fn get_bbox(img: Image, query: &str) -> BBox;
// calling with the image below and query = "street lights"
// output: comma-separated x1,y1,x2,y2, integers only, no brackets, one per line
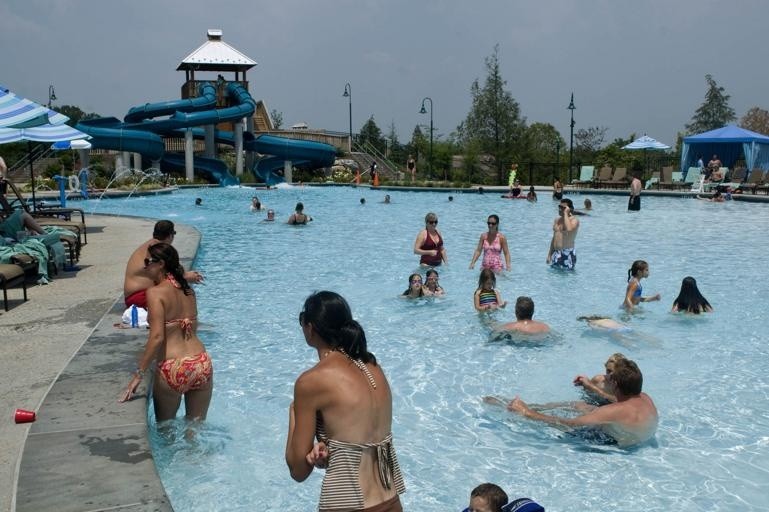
48,84,58,106
566,92,576,185
343,83,352,154
418,97,433,181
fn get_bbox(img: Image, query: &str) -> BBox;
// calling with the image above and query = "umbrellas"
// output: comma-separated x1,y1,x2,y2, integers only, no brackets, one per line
620,133,673,188
0,85,95,217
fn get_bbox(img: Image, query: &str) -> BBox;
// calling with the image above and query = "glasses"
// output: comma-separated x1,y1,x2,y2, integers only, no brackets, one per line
144,258,157,267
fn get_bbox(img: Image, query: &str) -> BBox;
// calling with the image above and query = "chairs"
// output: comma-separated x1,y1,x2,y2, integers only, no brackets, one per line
0,176,89,314
569,164,769,196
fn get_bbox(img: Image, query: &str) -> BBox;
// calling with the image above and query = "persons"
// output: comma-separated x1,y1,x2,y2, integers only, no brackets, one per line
550,175,564,201
501,161,539,203
576,311,656,354
1,156,14,213
384,194,391,203
400,195,599,341
119,214,215,444
463,481,511,512
627,172,643,212
194,198,202,205
406,153,417,182
619,259,662,316
2,204,49,242
478,353,660,454
670,273,716,317
477,186,484,195
247,197,313,226
283,288,409,512
695,153,735,203
369,161,379,186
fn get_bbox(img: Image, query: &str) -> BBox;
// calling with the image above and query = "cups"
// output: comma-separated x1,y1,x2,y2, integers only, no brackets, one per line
14,409,36,422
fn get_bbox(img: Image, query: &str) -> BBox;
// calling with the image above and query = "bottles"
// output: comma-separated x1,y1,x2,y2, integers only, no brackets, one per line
131,305,139,327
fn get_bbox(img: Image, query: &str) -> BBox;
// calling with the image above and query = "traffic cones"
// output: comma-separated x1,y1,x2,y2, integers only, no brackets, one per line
374,173,379,186
